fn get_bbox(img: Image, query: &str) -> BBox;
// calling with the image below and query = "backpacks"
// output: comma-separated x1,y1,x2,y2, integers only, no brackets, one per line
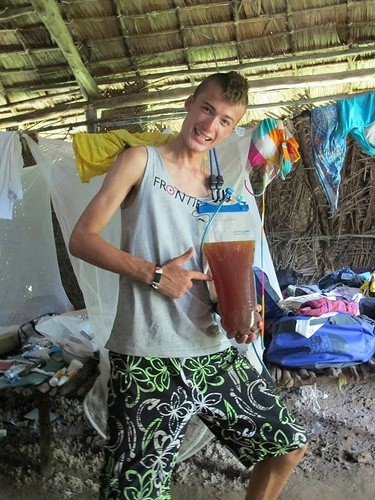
254,267,375,368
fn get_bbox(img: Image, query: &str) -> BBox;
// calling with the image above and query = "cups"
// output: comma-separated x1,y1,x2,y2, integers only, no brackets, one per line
67,359,84,373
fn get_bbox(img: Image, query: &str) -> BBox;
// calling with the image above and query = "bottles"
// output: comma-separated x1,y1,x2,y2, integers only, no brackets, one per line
57,369,78,387
49,367,66,386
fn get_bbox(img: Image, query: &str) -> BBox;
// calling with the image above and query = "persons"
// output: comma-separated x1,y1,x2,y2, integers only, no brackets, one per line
67,70,313,500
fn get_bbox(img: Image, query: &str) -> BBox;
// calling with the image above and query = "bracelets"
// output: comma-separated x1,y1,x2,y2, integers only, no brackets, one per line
149,264,162,290
